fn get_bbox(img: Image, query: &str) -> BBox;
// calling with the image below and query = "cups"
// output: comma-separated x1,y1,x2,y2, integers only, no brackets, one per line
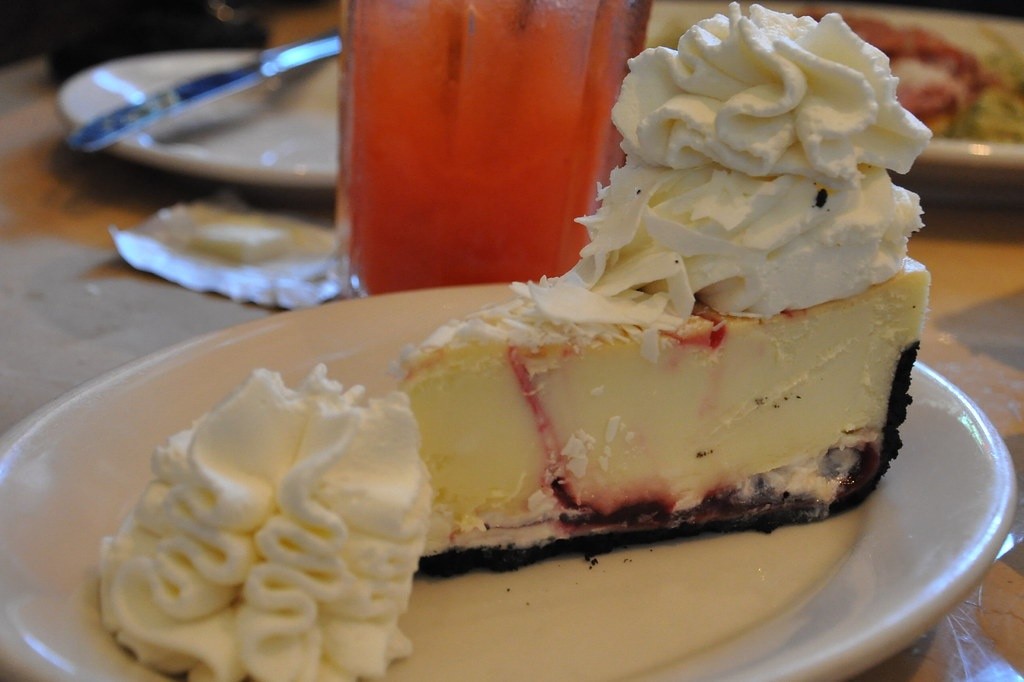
340,0,642,296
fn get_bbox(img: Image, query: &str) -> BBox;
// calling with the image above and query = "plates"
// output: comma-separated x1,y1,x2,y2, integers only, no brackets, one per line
647,0,1024,168
58,52,341,187
0,284,1015,682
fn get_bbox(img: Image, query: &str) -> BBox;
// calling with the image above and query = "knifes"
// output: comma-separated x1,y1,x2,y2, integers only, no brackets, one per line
68,34,341,153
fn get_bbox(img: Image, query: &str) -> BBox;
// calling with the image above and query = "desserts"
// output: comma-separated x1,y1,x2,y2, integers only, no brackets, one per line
97,1,933,682
